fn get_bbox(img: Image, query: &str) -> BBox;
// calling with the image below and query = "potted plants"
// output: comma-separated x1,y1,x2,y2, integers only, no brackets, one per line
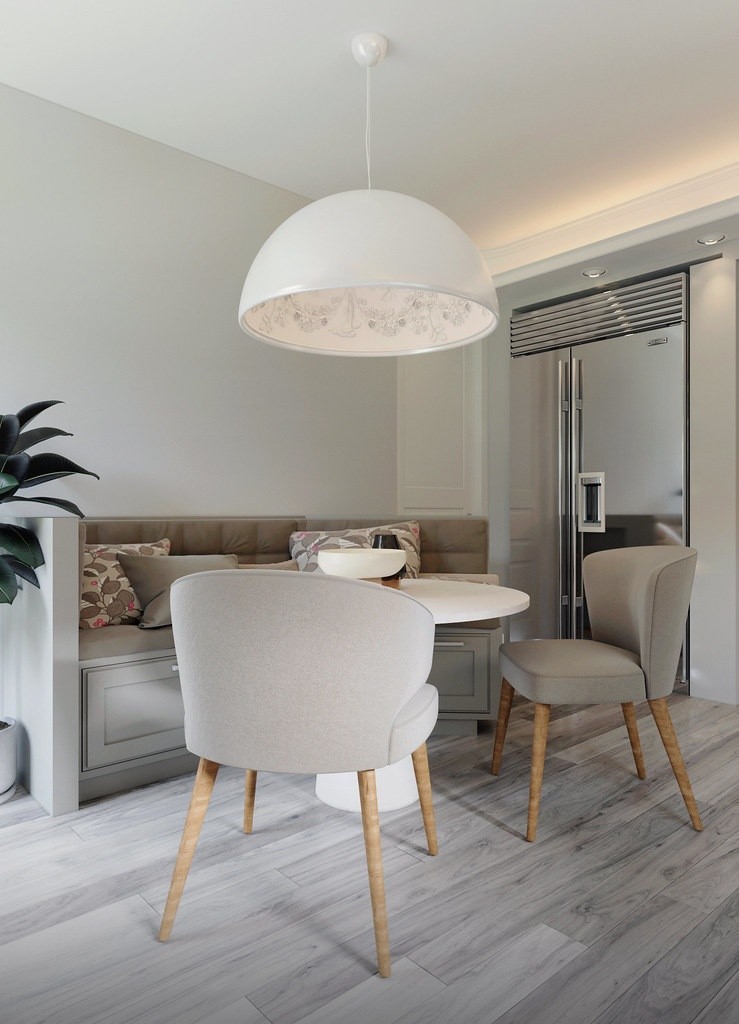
0,400,100,807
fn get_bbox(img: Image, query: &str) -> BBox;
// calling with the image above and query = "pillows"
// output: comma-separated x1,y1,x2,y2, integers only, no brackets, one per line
238,558,299,570
289,519,421,580
79,537,171,630
116,553,239,630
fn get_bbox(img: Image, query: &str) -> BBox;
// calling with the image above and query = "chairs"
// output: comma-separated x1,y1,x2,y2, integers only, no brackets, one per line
154,568,437,977
490,546,704,843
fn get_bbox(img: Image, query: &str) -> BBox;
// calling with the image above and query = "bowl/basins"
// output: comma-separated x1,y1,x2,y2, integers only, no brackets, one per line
317,547,408,579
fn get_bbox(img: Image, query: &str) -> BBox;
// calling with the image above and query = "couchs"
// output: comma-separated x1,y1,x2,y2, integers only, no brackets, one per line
15,514,503,817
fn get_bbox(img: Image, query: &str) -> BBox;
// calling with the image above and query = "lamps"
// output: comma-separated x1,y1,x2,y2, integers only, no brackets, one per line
237,34,500,357
